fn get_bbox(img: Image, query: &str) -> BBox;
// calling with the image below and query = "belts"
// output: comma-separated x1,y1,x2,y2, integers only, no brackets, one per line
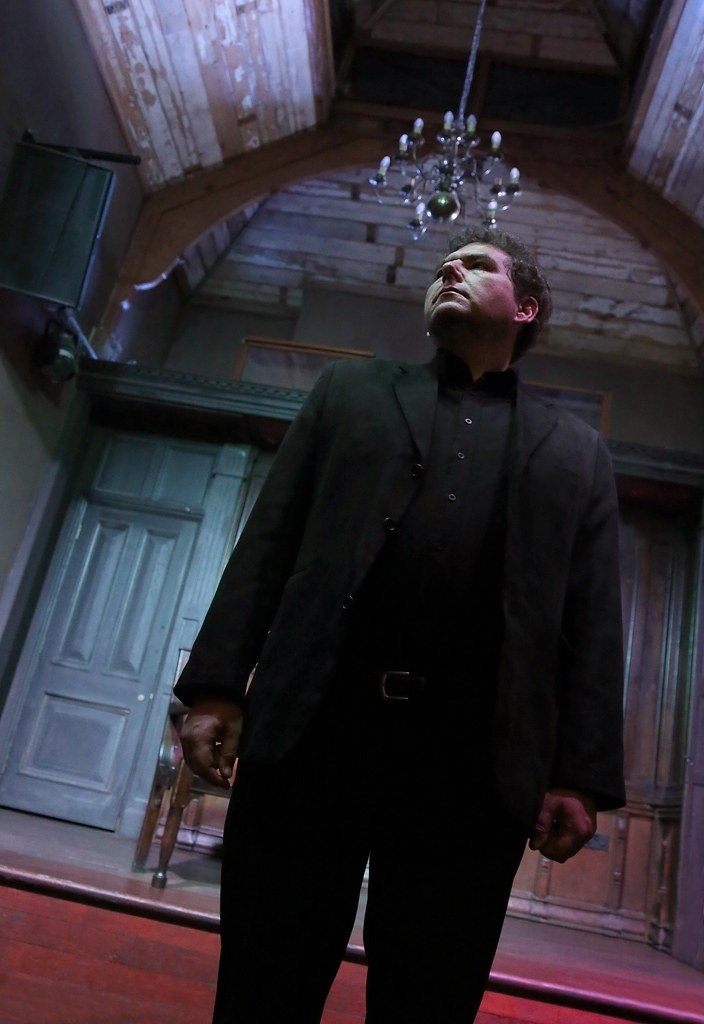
326,667,495,706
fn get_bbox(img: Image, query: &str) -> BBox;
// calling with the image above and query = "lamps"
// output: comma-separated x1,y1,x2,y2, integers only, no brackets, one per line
366,0,525,239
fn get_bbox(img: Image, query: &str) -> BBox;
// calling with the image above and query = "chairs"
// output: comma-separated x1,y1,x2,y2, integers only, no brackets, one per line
129,647,234,890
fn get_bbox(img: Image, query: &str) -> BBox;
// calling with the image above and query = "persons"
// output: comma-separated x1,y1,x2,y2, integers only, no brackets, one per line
174,229,626,1023
